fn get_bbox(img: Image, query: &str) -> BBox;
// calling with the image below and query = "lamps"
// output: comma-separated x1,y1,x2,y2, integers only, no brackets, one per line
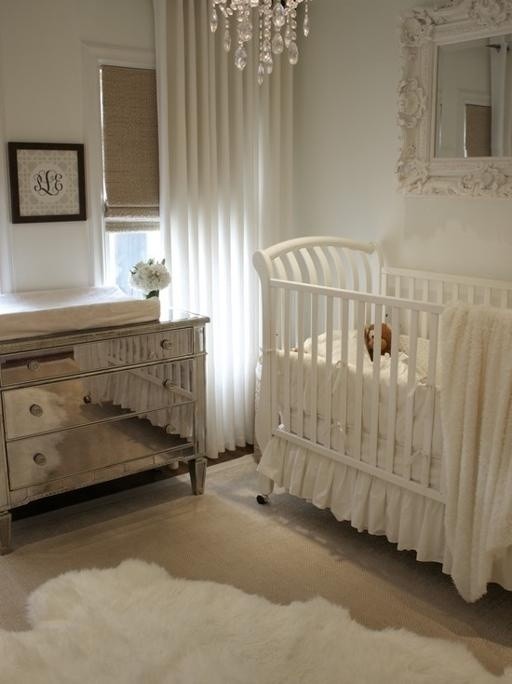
204,0,312,89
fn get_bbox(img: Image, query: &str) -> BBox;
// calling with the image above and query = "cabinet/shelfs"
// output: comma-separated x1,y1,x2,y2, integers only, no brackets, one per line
1,308,207,554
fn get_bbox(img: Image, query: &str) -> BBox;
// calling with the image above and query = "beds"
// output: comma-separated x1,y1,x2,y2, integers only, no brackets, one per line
251,238,511,601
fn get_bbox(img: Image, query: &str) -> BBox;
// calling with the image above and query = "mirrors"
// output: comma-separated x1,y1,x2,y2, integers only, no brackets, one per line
396,0,511,202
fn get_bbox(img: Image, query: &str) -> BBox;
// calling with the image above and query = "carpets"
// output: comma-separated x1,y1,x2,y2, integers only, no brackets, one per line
0,494,512,684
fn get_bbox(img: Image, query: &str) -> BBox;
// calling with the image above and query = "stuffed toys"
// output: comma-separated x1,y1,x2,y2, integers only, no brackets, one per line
366,323,422,384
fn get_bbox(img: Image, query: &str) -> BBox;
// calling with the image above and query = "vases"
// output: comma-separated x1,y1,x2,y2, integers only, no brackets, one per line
146,291,159,301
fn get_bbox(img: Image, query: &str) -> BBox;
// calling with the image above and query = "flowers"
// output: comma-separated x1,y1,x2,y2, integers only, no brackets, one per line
130,256,171,296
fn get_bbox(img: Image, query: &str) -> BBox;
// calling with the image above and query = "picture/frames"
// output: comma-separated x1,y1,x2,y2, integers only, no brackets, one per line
8,143,86,225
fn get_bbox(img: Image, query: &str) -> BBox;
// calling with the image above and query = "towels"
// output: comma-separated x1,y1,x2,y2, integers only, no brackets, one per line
433,301,511,601
